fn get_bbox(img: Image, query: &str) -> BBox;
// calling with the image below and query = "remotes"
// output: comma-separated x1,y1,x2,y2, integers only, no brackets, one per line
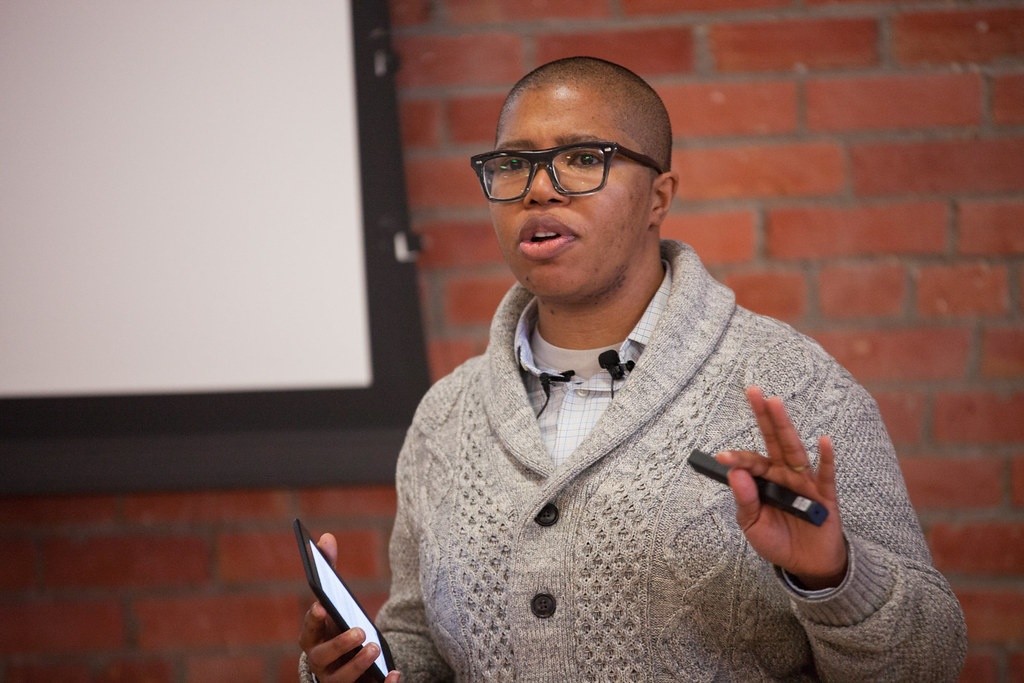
687,448,829,526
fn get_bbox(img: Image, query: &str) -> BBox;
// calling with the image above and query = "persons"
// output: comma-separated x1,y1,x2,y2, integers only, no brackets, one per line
300,55,970,683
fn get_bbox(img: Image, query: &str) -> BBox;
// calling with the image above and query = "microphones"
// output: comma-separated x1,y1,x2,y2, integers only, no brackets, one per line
598,349,625,380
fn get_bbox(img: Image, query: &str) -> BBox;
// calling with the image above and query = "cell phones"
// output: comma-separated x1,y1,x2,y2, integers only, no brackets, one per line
293,518,395,683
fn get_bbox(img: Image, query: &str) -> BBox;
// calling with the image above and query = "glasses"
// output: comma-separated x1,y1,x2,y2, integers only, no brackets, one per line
470,142,666,204
